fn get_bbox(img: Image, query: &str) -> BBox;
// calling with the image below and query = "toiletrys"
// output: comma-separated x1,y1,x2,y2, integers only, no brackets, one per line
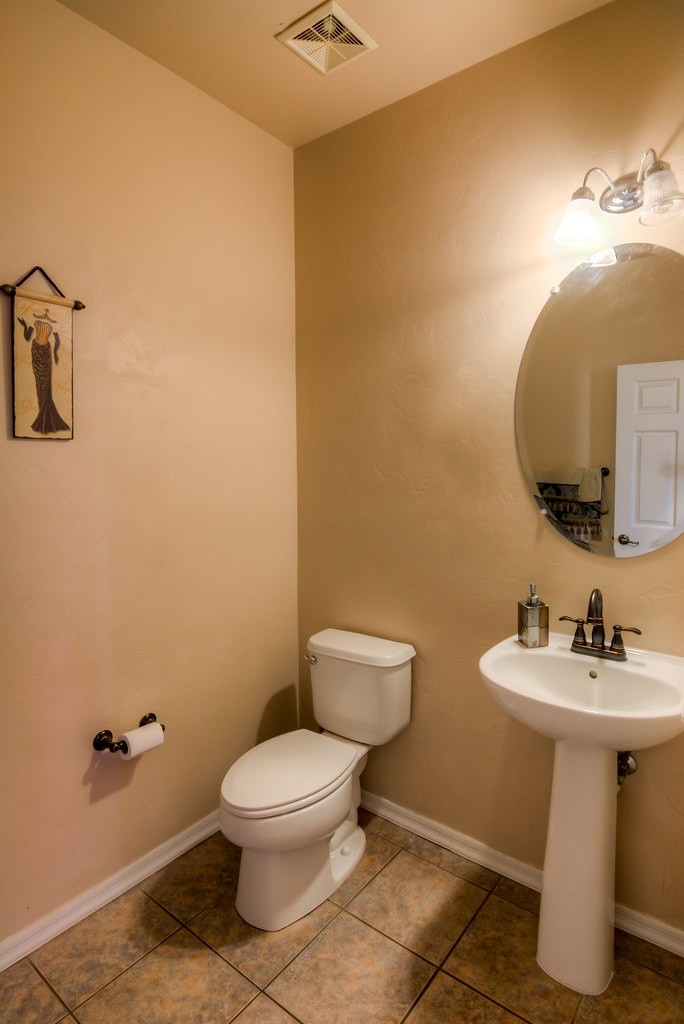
518,584,549,648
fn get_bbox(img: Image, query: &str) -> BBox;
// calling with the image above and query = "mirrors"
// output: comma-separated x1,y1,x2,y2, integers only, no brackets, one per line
513,242,683,560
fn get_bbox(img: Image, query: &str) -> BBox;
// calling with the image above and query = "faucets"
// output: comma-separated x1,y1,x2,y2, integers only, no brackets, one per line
557,588,641,662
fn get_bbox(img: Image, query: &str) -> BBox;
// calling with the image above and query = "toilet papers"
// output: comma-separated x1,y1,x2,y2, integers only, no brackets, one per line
119,721,165,759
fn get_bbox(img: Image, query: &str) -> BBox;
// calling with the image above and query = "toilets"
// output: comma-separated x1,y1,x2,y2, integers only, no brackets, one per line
219,630,415,931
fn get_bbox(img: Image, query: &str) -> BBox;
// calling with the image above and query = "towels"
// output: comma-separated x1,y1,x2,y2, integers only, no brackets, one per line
578,467,610,515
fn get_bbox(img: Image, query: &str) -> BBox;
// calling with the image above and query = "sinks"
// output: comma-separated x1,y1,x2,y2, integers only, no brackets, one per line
478,629,684,750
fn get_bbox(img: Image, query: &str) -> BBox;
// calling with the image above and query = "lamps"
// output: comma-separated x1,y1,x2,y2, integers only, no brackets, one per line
552,148,684,246
